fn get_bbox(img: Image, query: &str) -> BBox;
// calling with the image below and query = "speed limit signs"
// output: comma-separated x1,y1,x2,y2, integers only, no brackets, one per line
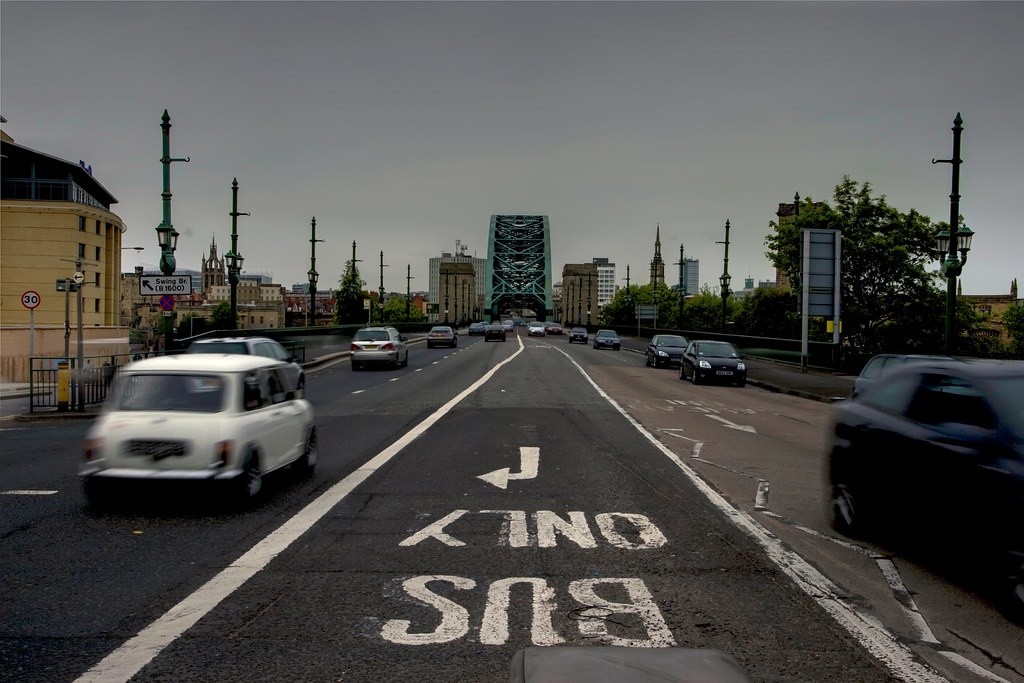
22,291,41,309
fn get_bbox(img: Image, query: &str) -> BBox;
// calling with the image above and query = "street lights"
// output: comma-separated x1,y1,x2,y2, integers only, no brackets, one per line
676,241,685,329
577,278,583,326
225,178,248,332
570,282,575,325
653,258,662,330
442,267,450,324
623,263,633,327
718,219,731,333
564,286,571,326
454,274,459,326
378,249,389,322
586,271,592,327
348,240,362,319
460,280,467,324
305,214,325,328
466,284,473,320
404,264,414,322
785,192,803,343
154,107,181,354
931,112,976,355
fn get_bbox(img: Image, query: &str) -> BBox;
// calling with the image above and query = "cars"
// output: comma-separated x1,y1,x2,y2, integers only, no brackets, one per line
427,325,457,349
545,322,563,336
845,353,967,400
483,324,507,342
819,360,1024,630
678,340,748,388
187,334,309,401
469,319,528,336
644,335,690,367
527,321,545,336
78,351,320,508
593,329,621,351
349,325,410,370
567,327,589,345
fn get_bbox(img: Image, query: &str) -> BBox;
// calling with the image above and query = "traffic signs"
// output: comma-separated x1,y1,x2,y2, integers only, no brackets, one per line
137,274,193,295
